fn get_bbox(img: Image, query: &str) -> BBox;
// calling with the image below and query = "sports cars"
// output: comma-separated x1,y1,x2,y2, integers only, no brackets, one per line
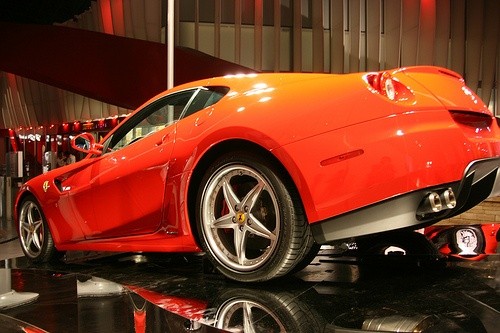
424,224,500,261
68,269,323,333
11,64,500,286
